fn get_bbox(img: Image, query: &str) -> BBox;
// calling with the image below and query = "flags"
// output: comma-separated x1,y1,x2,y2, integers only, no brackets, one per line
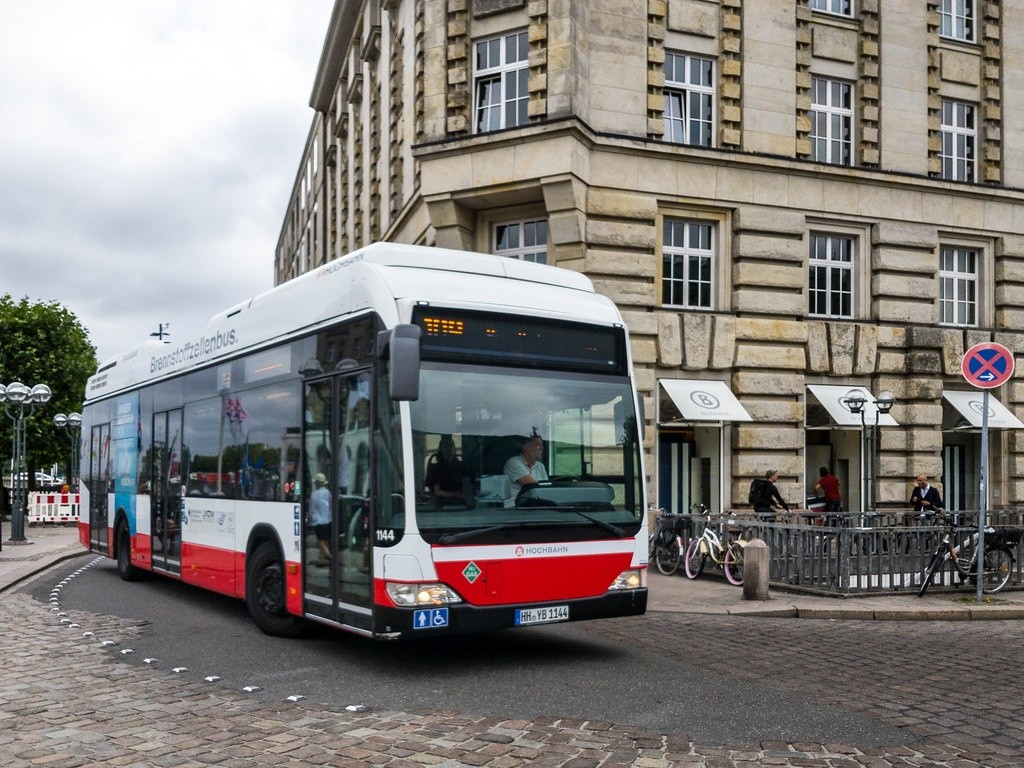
236,397,263,438
226,398,239,439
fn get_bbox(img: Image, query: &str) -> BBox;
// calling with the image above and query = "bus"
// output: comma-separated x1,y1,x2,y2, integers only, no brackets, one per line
80,241,649,641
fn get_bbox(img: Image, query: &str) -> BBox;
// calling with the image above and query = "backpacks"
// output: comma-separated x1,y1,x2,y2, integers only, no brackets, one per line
748,478,765,504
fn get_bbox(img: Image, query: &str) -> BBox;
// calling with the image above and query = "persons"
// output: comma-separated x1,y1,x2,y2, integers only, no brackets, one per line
307,472,333,561
432,436,473,497
814,466,842,527
502,433,549,508
909,474,943,521
753,469,793,522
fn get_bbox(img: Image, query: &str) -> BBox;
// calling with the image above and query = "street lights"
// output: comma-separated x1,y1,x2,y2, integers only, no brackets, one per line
0,382,52,546
843,390,895,555
53,412,82,493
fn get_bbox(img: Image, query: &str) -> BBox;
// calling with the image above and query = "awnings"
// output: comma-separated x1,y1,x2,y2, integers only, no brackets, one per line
657,378,754,422
805,385,901,426
942,390,1024,428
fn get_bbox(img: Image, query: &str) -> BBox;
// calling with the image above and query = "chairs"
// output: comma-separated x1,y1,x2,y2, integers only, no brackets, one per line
425,423,524,506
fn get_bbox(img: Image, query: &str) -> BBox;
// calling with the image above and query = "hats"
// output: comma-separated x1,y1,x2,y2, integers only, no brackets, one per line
765,468,777,480
312,473,328,485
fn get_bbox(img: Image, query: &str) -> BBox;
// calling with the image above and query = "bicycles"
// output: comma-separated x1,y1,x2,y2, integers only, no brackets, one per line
649,508,691,576
685,502,748,586
917,505,1016,598
